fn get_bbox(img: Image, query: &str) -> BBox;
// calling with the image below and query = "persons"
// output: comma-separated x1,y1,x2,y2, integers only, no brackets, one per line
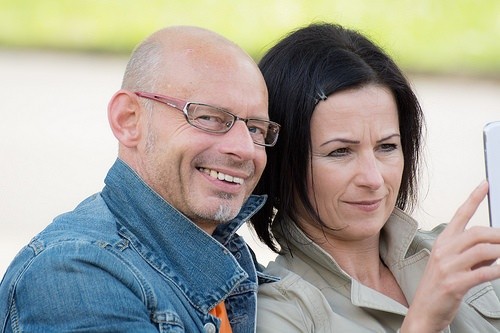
254,19,500,333
0,25,280,333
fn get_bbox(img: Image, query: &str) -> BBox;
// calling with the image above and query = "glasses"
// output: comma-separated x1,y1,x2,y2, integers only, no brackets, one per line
131,88,282,149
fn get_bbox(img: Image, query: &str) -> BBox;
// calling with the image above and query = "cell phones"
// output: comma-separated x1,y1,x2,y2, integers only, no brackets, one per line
483,121,500,231
